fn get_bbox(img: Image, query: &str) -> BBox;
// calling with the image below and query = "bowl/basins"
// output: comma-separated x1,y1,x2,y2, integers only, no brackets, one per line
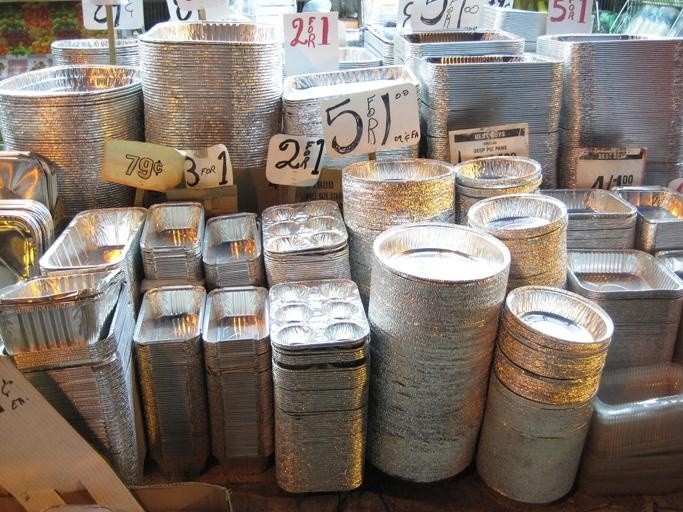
475,285,613,504
39,206,146,315
454,155,542,226
204,287,273,466
140,202,204,281
133,285,211,473
202,212,263,288
343,158,457,316
366,221,511,483
468,193,567,289
0,269,145,484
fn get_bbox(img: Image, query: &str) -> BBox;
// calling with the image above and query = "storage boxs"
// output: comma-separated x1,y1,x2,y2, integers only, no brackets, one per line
0,482,232,512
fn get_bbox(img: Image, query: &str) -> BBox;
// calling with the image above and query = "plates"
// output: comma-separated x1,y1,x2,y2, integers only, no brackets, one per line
569,247,683,367
0,151,63,237
586,365,683,496
140,21,284,169
284,64,422,168
365,27,394,66
542,188,637,249
261,200,350,288
52,37,140,67
0,62,145,230
394,27,526,157
340,47,382,71
267,280,370,493
479,4,548,52
613,185,682,253
657,250,682,280
421,53,565,188
0,199,53,286
537,33,683,190
